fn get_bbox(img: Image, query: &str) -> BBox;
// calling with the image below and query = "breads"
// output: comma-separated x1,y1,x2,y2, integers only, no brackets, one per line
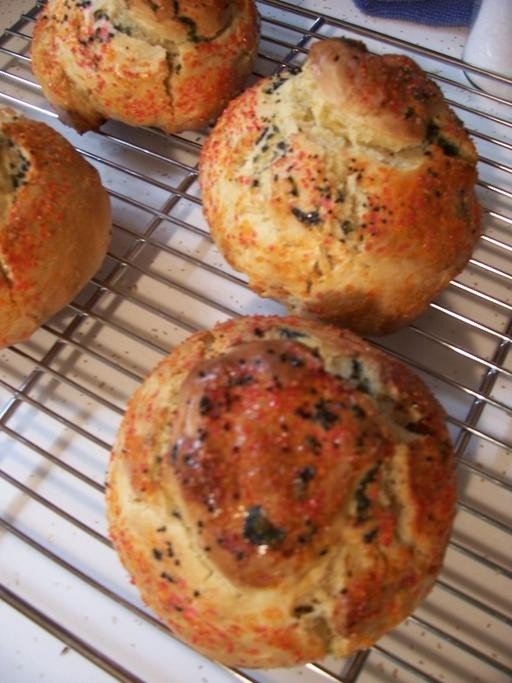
0,105,112,351
31,0,261,136
199,39,485,337
106,314,457,668
